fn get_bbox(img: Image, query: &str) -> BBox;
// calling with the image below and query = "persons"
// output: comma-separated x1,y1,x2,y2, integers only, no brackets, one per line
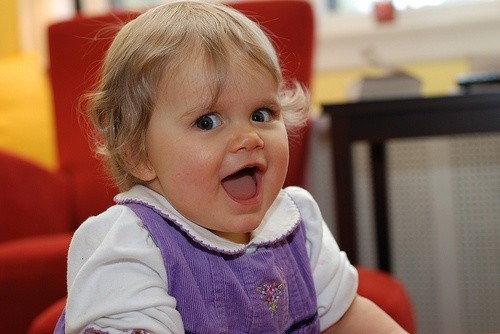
53,0,414,334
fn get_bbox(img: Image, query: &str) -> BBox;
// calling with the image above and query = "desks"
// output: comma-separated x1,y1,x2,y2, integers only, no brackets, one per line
320,92,500,275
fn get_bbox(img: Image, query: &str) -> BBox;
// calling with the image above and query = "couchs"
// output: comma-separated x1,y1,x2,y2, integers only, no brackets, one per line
0,1,416,334
